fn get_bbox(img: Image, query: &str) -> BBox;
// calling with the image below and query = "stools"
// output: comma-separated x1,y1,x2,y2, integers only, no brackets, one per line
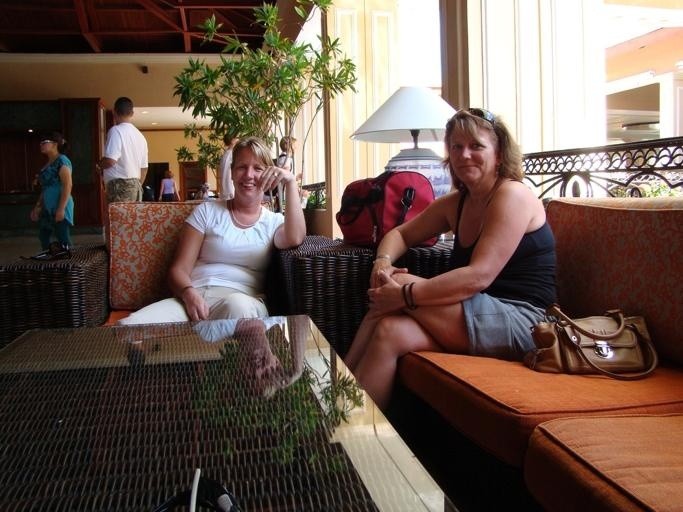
0,243,109,352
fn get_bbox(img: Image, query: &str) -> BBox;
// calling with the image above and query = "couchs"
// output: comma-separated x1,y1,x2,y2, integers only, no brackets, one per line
104,200,339,328
289,194,682,512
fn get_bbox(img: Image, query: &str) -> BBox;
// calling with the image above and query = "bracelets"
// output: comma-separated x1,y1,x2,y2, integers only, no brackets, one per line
402,283,418,311
409,281,416,306
375,255,391,260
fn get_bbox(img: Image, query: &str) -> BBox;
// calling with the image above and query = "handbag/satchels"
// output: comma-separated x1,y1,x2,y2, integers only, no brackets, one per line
334,169,440,249
527,302,659,382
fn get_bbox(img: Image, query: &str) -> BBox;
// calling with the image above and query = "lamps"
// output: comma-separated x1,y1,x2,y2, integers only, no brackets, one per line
348,84,456,243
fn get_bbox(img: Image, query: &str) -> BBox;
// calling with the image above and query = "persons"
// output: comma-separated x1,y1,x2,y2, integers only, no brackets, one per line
94,95,149,204
127,341,147,364
114,136,308,328
340,107,557,407
141,134,296,202
29,131,76,253
193,315,310,398
